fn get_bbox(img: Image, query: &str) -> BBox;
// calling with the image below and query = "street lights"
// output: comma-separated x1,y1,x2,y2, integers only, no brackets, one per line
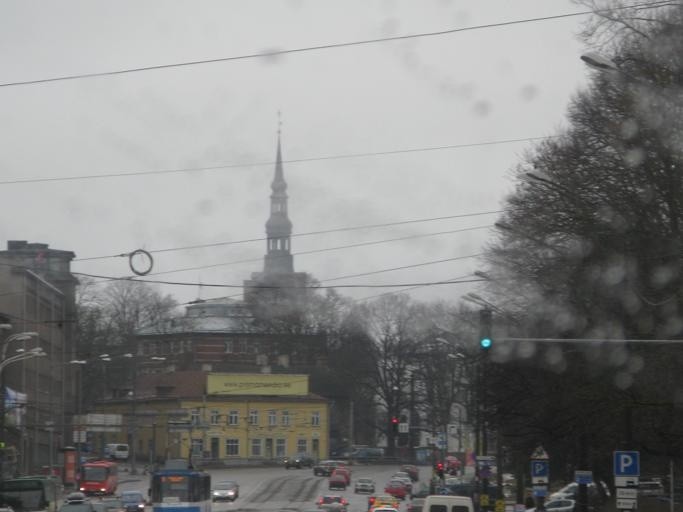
0,323,168,421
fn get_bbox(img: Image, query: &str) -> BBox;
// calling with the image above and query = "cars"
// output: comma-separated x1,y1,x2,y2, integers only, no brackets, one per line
327,468,350,489
523,478,609,511
56,490,146,511
282,452,315,468
444,455,460,469
211,480,239,501
314,494,349,512
354,478,376,493
163,458,194,469
367,464,424,511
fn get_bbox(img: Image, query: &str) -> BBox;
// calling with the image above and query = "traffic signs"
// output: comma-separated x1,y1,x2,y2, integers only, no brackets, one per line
474,454,497,462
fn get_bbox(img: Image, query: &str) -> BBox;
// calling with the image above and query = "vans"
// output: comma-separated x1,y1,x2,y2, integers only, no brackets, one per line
422,495,473,512
637,482,664,496
38,466,60,476
330,442,384,462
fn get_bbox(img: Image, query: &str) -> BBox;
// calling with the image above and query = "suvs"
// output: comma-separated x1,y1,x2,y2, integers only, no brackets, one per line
312,460,344,476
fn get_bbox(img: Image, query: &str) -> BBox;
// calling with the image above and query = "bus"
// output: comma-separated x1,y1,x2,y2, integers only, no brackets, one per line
148,468,211,511
102,442,130,459
78,460,117,497
0,473,60,512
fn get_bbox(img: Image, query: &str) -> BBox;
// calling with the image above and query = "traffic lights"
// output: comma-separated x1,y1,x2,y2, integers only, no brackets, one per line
432,461,445,483
389,413,408,434
